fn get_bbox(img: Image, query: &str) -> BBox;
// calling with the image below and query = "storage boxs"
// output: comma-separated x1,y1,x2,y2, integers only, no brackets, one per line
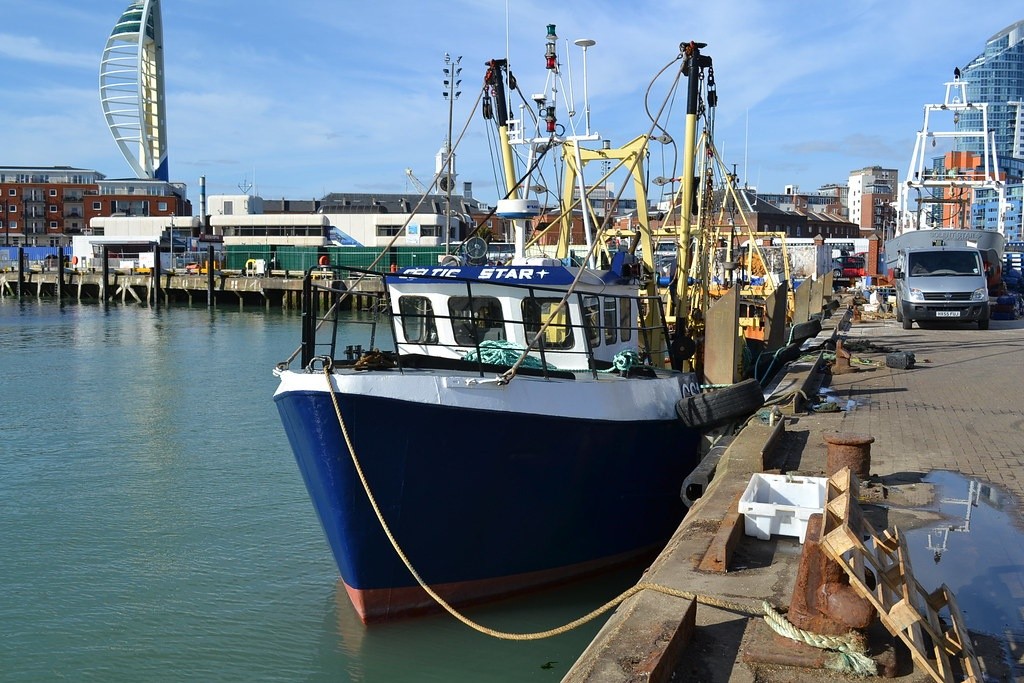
738,473,828,545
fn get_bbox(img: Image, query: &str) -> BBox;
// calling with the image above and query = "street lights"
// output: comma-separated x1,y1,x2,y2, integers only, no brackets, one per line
442,53,462,255
602,161,611,220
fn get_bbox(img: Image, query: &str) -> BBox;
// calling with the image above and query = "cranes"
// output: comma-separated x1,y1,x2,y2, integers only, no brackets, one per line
406,168,433,195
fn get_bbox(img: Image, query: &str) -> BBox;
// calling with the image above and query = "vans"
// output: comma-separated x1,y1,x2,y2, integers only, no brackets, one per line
893,246,990,330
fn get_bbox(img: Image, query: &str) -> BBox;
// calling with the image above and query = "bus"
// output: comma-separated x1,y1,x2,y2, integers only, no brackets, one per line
740,238,870,262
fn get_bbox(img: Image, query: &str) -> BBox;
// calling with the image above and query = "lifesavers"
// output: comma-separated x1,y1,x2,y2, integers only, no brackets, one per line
319,255,330,265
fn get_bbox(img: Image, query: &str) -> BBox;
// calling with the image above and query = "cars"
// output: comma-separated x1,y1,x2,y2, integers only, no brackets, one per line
654,241,677,259
832,256,865,278
840,252,869,276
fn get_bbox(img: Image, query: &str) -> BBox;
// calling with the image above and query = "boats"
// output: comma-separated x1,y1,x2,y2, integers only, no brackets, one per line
886,67,1006,287
272,23,795,624
